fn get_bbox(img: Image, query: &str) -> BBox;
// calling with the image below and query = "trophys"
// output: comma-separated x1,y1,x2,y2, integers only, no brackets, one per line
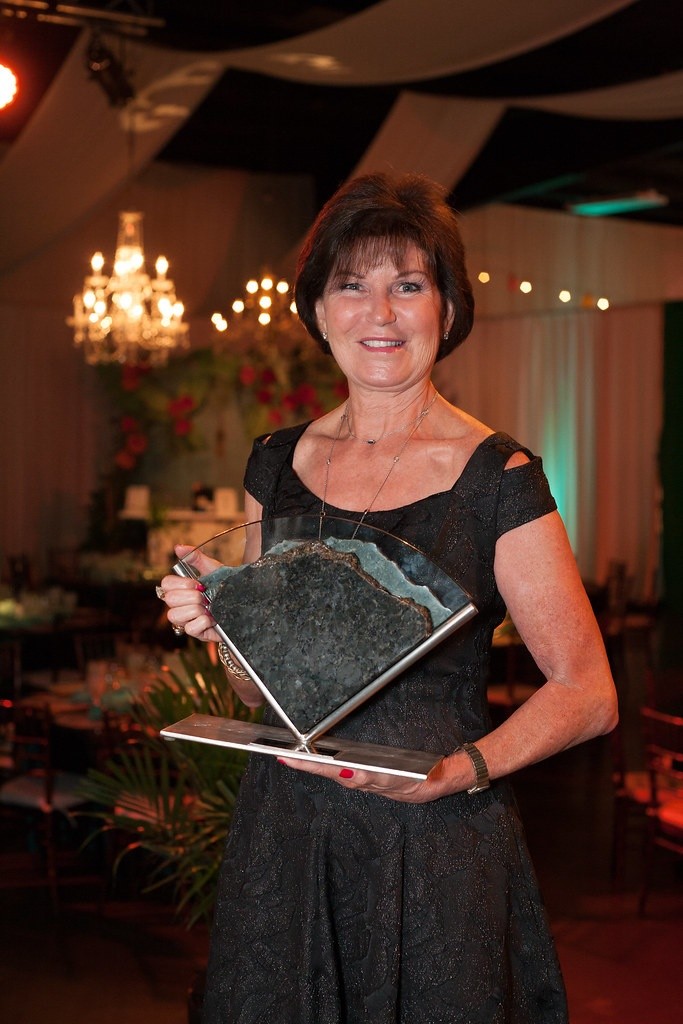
160,514,480,781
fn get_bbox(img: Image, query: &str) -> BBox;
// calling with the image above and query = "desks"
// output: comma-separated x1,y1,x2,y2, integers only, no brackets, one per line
24,681,256,802
0,607,110,688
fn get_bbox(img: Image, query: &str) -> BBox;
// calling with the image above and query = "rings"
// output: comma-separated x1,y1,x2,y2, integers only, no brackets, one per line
155,586,166,604
171,623,186,636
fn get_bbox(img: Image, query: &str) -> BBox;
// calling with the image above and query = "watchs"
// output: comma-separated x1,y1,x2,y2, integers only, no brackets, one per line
452,742,491,795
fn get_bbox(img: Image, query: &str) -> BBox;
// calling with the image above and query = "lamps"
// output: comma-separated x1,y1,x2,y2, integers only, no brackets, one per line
69,111,191,368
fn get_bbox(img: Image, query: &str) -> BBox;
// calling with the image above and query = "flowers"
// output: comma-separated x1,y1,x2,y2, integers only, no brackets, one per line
112,366,195,474
238,368,348,425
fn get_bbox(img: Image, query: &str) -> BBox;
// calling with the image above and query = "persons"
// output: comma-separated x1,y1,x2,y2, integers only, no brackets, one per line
159,173,621,1023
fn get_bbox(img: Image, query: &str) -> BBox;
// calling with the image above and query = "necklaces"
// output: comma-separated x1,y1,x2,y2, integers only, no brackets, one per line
317,390,439,543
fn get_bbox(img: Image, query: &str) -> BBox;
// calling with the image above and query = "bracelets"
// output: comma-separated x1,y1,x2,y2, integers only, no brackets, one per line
216,641,253,682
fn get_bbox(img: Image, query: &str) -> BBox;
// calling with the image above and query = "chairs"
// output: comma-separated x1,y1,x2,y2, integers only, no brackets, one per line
636,706,683,922
0,700,101,878
615,600,657,667
9,555,32,598
